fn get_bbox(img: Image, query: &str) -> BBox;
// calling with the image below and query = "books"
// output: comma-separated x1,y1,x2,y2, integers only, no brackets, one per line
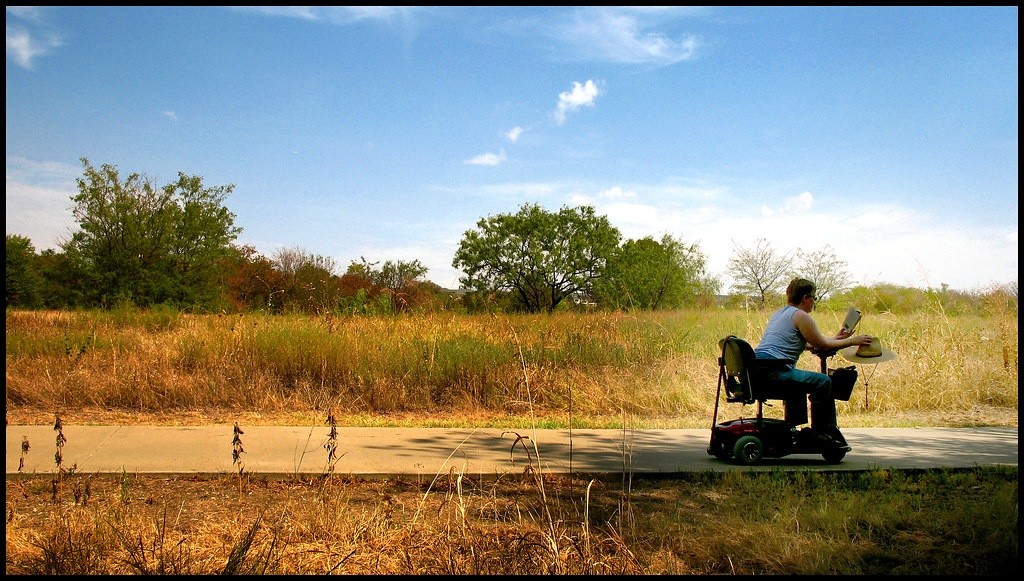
842,309,862,333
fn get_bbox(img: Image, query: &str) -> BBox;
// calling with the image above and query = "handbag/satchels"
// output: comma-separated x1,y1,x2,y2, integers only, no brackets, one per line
830,366,859,401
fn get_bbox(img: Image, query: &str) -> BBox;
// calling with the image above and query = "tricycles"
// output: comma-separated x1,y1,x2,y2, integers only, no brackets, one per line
708,336,849,464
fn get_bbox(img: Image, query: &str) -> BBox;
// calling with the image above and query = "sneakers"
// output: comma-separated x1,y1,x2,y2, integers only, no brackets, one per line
816,432,842,448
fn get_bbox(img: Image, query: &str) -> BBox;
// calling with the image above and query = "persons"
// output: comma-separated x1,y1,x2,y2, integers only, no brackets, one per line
753,279,873,445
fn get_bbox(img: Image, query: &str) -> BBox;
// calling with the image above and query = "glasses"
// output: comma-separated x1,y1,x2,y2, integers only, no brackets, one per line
804,293,819,304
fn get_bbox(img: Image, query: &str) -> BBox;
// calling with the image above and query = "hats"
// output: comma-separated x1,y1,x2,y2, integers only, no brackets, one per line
841,337,898,364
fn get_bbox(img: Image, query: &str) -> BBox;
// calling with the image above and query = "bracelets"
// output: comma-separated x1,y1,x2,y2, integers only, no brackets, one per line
850,338,853,344
